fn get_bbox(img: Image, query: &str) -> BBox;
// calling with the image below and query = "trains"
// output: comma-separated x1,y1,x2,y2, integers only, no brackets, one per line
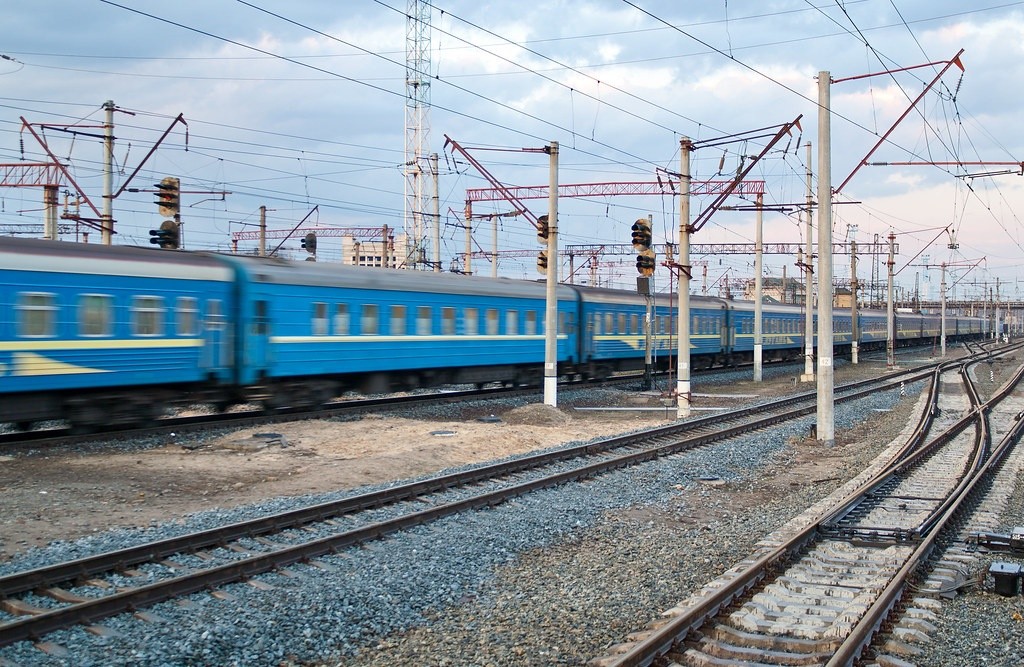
1,232,1007,437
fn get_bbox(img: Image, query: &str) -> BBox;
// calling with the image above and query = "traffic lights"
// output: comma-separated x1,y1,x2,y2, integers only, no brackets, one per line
300,234,317,253
537,249,548,275
154,176,179,218
537,215,549,245
636,247,656,276
631,218,651,252
149,220,179,249
